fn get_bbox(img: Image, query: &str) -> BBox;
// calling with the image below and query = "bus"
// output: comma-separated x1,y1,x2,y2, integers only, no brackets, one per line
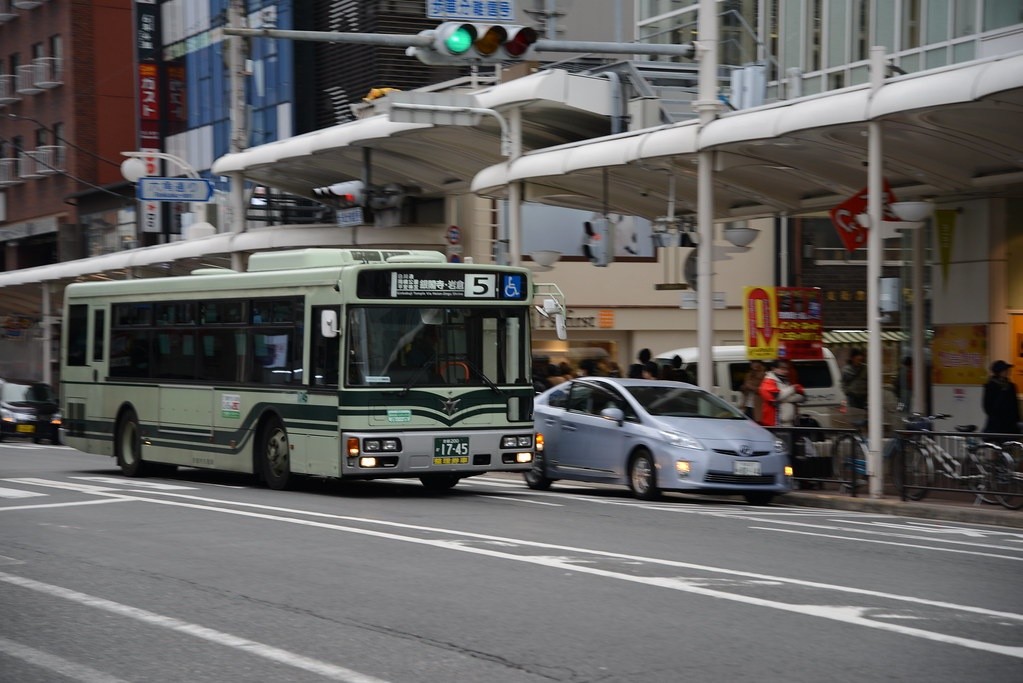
54,248,568,491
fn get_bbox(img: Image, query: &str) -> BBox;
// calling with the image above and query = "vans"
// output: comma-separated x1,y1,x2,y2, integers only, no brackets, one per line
643,343,851,479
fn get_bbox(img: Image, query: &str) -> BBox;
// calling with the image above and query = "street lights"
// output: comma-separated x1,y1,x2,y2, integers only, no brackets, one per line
116,147,218,238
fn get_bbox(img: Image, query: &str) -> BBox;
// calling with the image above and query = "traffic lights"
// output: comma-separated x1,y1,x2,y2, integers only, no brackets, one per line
581,217,617,268
432,20,540,63
312,179,364,205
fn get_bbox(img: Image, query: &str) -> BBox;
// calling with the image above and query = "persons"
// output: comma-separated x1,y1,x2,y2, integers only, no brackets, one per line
980,360,1023,447
740,357,807,463
411,325,441,388
840,348,868,410
24,373,65,446
533,348,696,397
893,356,914,413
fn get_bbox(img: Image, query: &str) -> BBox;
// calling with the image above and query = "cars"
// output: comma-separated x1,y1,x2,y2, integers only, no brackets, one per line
0,375,61,445
521,375,797,507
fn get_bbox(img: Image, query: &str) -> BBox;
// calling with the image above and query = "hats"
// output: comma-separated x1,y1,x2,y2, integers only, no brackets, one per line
992,360,1014,372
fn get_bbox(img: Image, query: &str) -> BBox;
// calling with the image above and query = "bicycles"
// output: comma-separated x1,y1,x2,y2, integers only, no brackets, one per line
826,400,1023,512
